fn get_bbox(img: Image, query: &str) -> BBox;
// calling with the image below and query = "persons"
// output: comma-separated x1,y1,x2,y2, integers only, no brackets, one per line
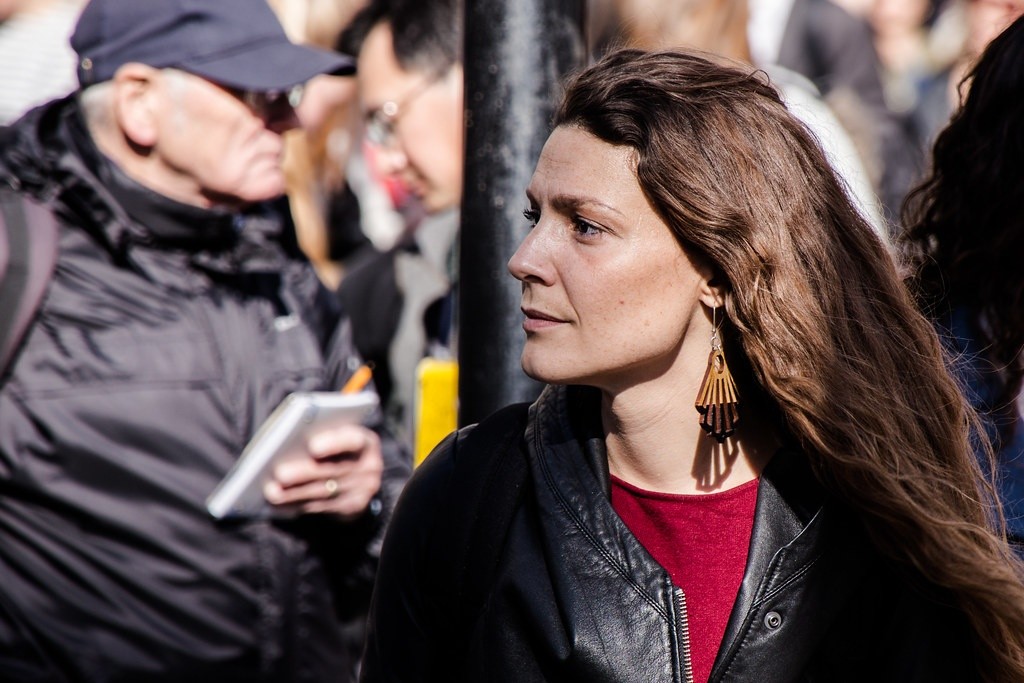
0,1,413,683
0,1,1024,573
353,44,1024,683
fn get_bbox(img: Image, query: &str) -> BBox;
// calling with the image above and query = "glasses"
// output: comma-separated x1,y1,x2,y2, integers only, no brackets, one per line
240,85,307,107
364,58,458,142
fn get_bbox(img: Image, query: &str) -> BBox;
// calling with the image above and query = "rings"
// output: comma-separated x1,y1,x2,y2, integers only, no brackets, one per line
324,479,339,496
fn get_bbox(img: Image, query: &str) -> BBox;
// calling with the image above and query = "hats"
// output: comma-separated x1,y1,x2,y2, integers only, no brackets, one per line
69,0,358,92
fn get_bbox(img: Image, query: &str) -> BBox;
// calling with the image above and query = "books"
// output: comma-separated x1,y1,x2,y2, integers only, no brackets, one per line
205,390,382,529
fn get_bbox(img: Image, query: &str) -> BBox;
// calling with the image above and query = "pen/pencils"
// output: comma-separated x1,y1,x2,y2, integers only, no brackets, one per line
340,365,371,392
